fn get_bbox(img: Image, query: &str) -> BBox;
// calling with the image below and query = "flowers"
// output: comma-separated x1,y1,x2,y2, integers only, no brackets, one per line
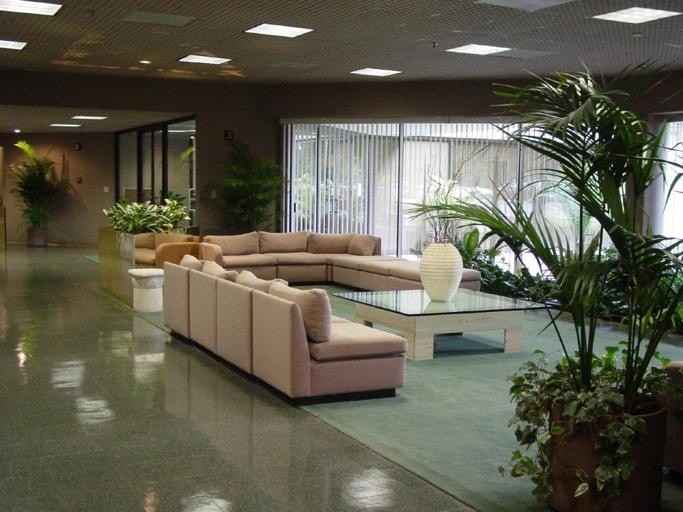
102,195,191,234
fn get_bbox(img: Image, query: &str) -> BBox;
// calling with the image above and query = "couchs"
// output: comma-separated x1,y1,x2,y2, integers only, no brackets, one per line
199,230,481,292
134,231,199,267
162,259,407,407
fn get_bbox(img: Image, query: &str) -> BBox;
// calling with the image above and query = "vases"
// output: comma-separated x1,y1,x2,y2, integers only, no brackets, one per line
420,242,463,302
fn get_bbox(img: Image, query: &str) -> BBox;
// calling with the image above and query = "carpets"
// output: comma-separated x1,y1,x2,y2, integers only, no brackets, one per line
84,253,683,512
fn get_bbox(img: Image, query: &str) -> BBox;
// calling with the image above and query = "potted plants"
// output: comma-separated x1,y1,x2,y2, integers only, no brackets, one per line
402,56,683,512
8,140,70,248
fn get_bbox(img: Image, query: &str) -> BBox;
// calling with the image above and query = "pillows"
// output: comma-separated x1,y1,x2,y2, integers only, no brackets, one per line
205,231,375,256
178,254,331,344
